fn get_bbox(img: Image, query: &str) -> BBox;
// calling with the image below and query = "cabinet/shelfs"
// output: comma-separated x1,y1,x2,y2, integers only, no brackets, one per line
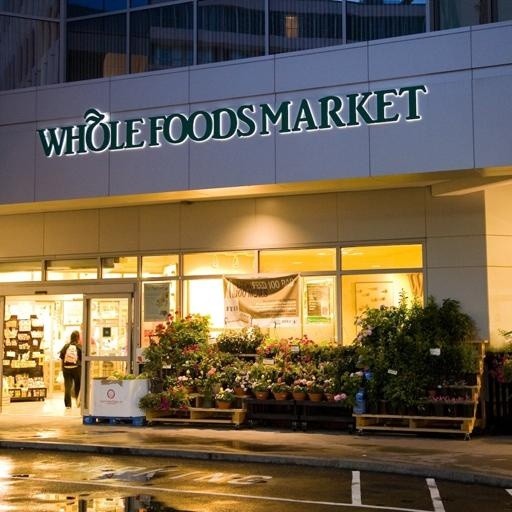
352,339,488,441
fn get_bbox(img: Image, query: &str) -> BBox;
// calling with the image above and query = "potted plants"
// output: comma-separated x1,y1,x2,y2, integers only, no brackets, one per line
361,297,476,428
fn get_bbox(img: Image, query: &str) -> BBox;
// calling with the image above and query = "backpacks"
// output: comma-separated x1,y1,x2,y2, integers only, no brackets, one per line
64,344,78,370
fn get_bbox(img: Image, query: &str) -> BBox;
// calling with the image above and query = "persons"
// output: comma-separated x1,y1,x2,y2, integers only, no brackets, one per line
58,329,82,408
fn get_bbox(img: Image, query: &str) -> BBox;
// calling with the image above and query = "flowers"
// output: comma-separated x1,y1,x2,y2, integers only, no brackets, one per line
136,312,358,424
